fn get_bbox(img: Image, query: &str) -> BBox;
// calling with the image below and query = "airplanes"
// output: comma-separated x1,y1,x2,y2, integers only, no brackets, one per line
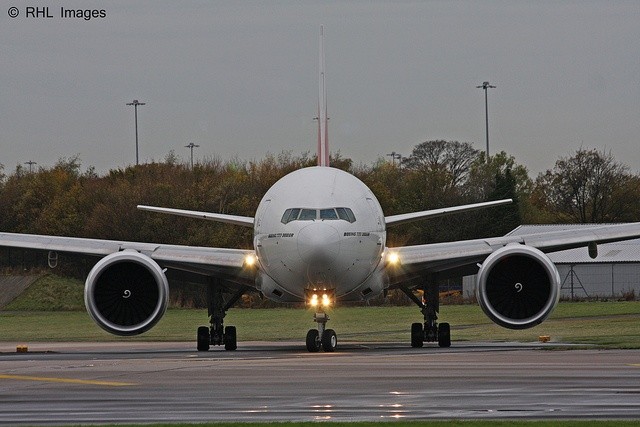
0,24,640,352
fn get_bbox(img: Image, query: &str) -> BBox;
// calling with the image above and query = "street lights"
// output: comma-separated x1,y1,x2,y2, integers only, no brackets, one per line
126,100,146,165
476,82,496,164
184,143,200,171
